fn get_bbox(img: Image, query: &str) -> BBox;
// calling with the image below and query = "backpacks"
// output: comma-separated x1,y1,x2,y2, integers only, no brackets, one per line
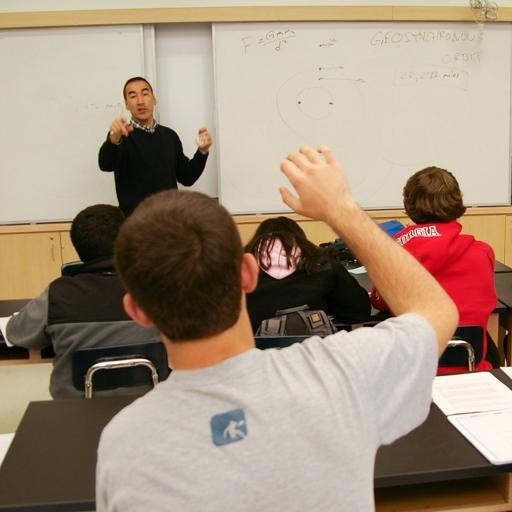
253,304,338,338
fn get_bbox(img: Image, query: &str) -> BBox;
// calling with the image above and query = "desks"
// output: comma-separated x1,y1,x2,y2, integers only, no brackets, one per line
0,259,512,512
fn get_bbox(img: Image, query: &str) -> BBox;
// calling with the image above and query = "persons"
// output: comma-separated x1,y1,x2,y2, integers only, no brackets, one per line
244,215,372,337
94,143,461,511
98,76,212,218
370,165,498,375
7,204,162,400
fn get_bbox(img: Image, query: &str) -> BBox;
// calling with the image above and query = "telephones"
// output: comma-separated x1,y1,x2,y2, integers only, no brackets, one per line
0,24,512,225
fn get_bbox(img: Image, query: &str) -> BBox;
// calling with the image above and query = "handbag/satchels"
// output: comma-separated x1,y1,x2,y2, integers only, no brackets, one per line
319,238,361,267
377,220,404,235
